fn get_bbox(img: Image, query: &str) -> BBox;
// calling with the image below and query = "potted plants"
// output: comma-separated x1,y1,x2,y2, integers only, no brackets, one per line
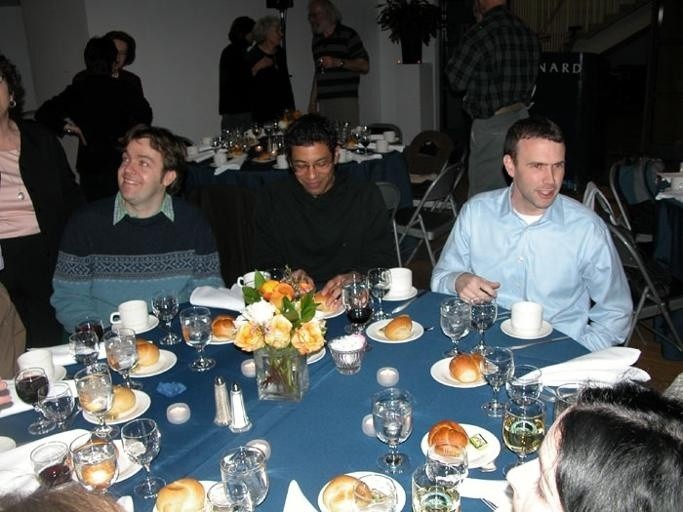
372,1,451,65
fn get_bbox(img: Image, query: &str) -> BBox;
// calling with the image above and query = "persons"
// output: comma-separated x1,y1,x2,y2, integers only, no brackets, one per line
48,123,228,343
246,14,296,123
444,0,542,200
25,36,155,207
217,15,257,138
247,111,399,286
1,55,84,355
431,115,635,354
496,382,682,512
100,28,153,121
307,0,371,124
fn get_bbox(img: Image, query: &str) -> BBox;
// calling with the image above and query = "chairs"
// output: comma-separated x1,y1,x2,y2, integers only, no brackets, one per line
400,159,469,270
364,123,403,146
403,128,460,213
601,219,682,364
374,179,400,271
586,153,682,345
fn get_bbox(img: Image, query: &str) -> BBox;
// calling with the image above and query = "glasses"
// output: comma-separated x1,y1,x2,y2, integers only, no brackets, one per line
288,152,334,175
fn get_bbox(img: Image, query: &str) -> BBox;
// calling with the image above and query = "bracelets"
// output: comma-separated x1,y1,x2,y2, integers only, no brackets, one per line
338,59,345,68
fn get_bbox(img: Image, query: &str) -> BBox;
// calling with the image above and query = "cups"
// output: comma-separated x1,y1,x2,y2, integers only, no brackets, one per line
355,473,398,512
205,444,270,511
409,465,461,512
181,137,210,161
108,301,150,332
327,266,411,377
236,271,270,289
372,131,399,154
511,300,542,335
425,443,469,490
672,175,683,192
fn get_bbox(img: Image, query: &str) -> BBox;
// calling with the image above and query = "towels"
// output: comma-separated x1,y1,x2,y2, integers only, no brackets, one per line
280,479,320,512
507,342,652,399
188,280,251,317
1,340,106,499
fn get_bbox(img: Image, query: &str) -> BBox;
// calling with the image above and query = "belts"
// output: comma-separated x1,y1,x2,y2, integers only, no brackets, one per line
495,102,523,115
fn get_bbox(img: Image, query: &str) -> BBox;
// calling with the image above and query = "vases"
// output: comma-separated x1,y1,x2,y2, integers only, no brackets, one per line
252,347,311,403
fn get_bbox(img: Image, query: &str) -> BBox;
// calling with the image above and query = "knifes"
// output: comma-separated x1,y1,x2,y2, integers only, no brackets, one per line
498,334,569,352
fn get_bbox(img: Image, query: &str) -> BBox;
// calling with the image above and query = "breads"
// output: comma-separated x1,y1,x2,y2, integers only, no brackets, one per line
428,419,469,457
136,337,159,368
313,293,336,312
78,439,119,488
210,314,234,338
450,352,489,382
155,478,206,512
78,383,137,414
384,316,414,341
322,475,371,512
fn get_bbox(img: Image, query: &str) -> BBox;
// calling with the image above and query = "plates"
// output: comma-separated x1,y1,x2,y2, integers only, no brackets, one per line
500,320,552,340
430,356,496,388
317,471,404,512
421,422,501,473
206,300,345,367
382,285,416,302
365,318,425,344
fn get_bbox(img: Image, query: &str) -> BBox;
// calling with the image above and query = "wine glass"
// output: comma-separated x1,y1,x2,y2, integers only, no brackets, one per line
372,388,411,474
180,307,216,371
210,118,366,169
14,312,166,498
438,297,472,359
467,297,497,357
151,288,182,346
482,347,587,477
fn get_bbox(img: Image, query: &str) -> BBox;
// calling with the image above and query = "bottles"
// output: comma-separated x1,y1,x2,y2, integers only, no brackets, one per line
210,375,229,433
227,381,252,435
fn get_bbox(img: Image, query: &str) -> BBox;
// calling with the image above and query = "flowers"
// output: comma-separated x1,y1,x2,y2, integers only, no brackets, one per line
228,265,328,392
